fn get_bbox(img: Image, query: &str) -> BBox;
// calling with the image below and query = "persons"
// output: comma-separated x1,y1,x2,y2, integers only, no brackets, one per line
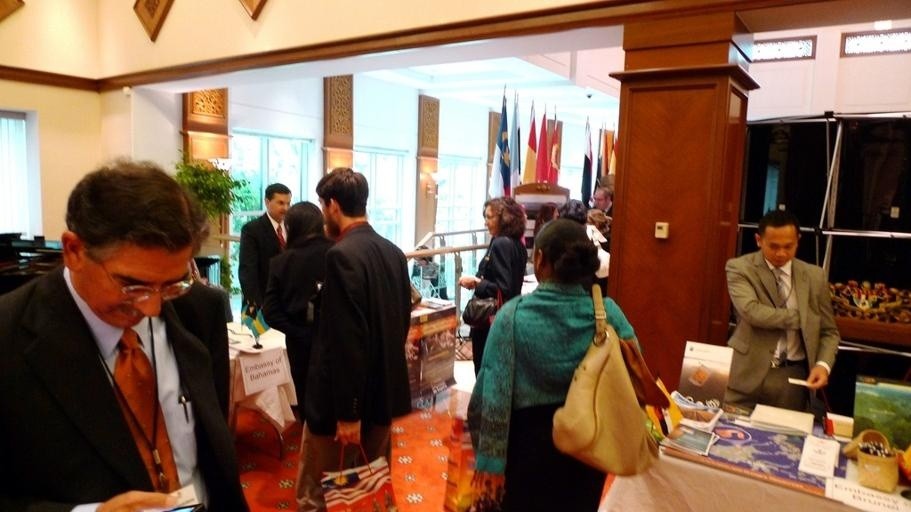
457,187,613,378
467,218,644,512
262,201,336,424
293,165,412,512
238,182,293,319
0,159,253,512
412,245,448,300
164,201,230,425
725,210,842,414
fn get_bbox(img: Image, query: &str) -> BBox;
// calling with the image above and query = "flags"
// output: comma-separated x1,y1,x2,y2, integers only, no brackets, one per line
488,95,618,208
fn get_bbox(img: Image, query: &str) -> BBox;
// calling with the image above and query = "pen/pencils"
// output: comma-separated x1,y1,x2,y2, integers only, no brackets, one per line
857,440,890,457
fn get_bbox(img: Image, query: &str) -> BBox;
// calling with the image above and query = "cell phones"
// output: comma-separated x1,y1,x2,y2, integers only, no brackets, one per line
164,504,202,512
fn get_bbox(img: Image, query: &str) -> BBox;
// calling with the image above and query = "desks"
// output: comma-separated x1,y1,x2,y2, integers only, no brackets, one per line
227,295,458,462
598,409,911,510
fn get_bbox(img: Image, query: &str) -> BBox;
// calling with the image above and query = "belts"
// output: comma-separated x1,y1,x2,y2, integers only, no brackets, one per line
772,356,808,368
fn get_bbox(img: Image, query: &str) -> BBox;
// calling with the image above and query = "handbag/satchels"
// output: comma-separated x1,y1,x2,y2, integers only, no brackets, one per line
550,322,660,476
462,295,504,327
319,455,400,512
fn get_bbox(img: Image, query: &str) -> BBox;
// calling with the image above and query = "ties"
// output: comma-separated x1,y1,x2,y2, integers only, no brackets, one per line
113,328,182,494
772,268,787,365
276,224,285,247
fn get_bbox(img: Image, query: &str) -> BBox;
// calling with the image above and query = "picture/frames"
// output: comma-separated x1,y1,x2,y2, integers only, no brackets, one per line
239,0,267,19
132,0,175,41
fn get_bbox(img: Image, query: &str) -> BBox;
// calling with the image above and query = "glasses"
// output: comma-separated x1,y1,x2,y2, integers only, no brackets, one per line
81,242,198,304
